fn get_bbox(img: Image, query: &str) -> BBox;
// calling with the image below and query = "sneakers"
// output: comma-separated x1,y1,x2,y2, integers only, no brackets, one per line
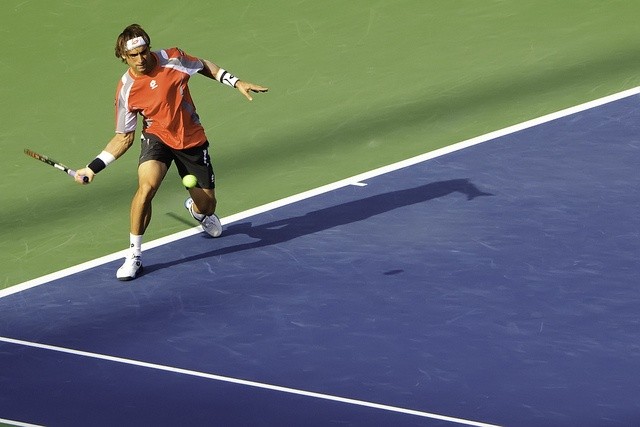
184,196,223,237
116,253,144,281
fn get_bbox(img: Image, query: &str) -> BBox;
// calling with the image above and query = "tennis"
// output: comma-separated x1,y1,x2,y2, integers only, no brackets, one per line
183,175,197,188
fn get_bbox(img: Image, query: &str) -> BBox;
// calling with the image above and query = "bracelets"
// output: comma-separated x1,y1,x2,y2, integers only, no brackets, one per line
88,158,106,173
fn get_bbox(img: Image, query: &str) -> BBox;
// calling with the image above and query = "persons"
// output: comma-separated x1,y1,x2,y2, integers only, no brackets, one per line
73,23,268,282
